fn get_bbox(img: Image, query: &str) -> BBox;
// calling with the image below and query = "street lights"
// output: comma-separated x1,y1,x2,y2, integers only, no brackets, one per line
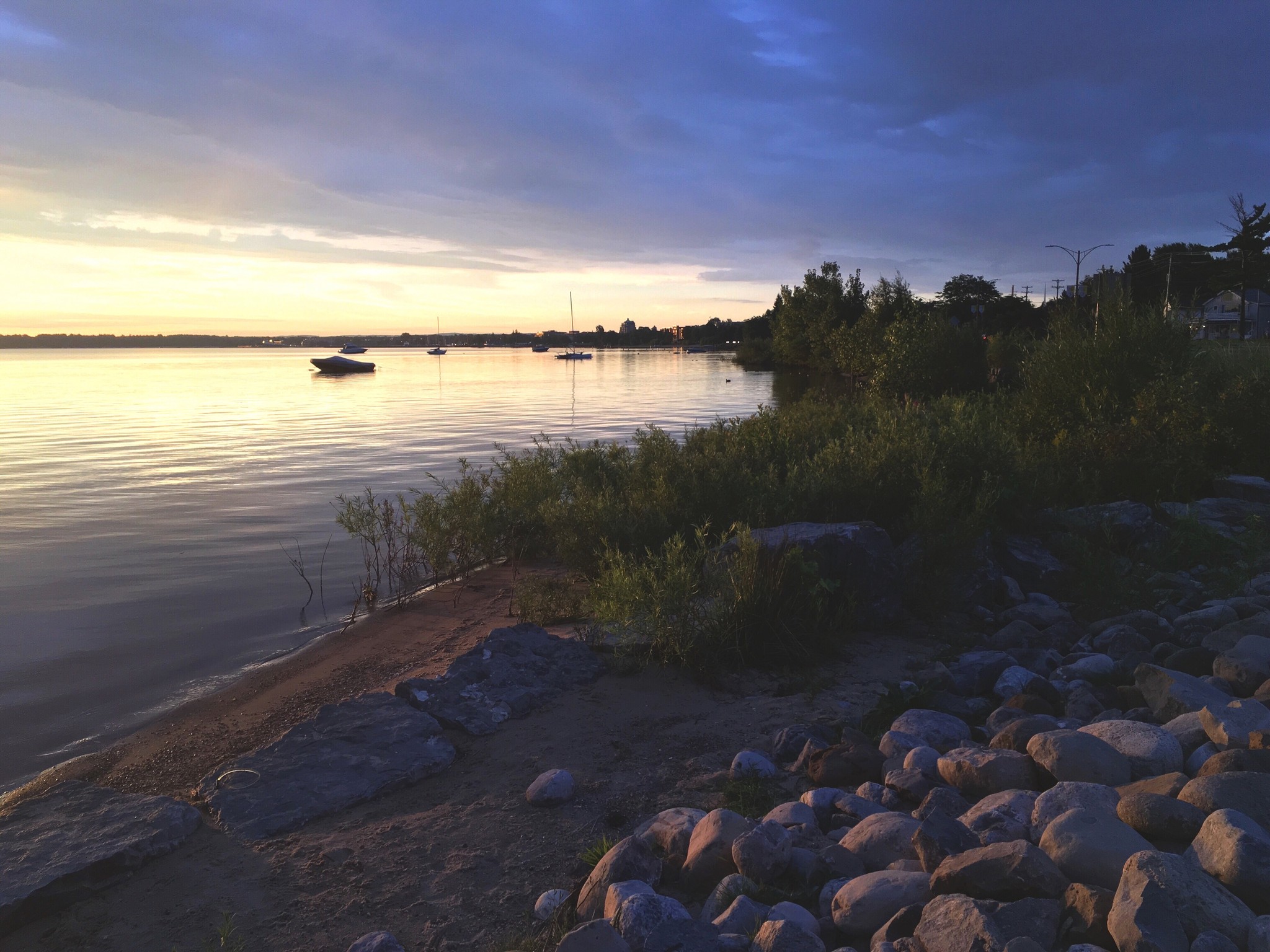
1044,244,1115,301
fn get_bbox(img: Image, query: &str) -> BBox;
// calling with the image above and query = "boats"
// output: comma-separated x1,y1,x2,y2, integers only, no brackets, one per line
338,342,368,354
308,356,375,371
531,344,549,352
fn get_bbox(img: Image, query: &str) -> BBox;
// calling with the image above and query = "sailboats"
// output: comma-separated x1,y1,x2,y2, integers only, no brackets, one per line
427,317,447,354
554,291,593,360
